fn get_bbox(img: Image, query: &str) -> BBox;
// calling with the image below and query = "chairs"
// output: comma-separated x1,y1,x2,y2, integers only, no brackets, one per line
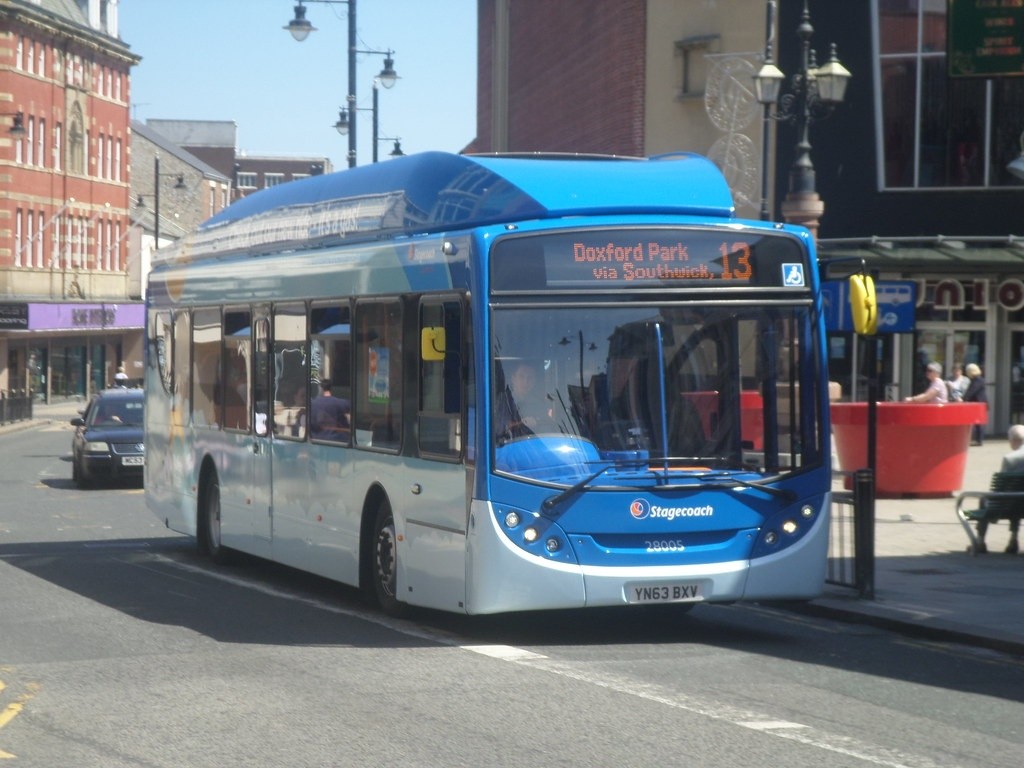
664,386,709,454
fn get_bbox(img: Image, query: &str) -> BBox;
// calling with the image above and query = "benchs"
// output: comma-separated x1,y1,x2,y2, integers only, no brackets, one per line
954,472,1024,557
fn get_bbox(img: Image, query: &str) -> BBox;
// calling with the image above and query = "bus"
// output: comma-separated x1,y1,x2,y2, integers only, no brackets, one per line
143,151,831,621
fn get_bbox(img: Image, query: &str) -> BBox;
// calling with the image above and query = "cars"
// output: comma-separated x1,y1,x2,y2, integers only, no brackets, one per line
70,386,144,490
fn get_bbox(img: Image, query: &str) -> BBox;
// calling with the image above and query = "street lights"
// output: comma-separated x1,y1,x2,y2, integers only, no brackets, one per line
331,77,408,163
130,151,190,250
281,1,398,169
750,0,851,473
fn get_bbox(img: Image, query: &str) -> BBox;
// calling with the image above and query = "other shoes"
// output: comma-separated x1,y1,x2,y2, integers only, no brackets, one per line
1005,539,1018,553
967,543,987,552
970,440,982,445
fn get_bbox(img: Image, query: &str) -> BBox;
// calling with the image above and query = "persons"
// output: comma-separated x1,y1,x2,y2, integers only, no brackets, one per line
279,384,307,428
311,379,352,444
114,367,129,389
495,350,648,445
903,361,990,446
967,424,1024,553
29,355,38,382
